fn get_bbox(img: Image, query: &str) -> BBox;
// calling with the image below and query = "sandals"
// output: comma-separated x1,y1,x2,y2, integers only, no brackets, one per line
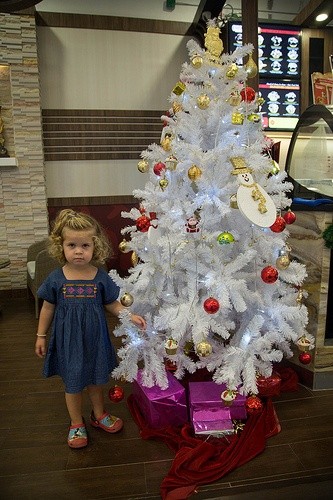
67,416,87,448
90,408,123,432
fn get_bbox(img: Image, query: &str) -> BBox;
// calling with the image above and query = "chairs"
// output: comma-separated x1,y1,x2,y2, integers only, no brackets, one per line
27,238,62,319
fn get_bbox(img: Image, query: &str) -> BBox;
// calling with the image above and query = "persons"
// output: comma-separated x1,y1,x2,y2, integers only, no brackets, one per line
33,208,148,451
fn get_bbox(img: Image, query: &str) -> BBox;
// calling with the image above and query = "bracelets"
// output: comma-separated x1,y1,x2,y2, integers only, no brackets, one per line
36,333,47,337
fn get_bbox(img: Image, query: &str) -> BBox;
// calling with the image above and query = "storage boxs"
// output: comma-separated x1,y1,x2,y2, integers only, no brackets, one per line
188,381,247,435
131,369,187,429
